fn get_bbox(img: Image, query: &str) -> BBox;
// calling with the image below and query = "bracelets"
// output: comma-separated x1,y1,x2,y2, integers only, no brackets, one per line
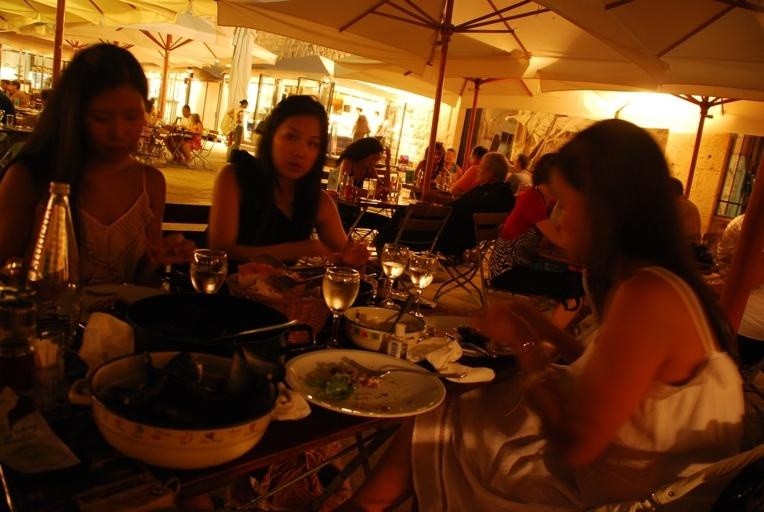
521,365,555,386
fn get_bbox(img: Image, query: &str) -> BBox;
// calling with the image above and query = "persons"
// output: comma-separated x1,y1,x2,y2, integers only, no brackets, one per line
420,150,517,266
330,137,399,250
196,92,373,273
1,43,205,291
325,117,745,511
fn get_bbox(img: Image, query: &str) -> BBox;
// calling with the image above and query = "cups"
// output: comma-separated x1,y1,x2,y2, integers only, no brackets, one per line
34,329,66,388
326,167,457,203
0,293,38,389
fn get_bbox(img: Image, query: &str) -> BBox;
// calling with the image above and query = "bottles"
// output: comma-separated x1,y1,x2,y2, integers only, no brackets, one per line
25,180,82,293
161,264,172,292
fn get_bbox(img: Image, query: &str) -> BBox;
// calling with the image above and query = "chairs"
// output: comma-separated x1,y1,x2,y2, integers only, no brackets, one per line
320,159,510,309
594,377,764,512
136,117,218,171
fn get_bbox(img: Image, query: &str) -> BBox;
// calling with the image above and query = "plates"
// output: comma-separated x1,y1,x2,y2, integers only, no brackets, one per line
286,350,456,420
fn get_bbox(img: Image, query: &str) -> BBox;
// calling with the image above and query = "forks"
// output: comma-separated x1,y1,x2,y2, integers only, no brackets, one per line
340,357,468,385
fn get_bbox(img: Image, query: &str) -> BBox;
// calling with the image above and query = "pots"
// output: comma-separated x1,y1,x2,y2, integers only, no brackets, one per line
66,347,294,472
106,293,313,367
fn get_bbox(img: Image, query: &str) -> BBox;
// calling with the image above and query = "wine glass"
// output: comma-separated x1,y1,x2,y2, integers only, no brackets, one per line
321,250,493,364
188,248,230,295
